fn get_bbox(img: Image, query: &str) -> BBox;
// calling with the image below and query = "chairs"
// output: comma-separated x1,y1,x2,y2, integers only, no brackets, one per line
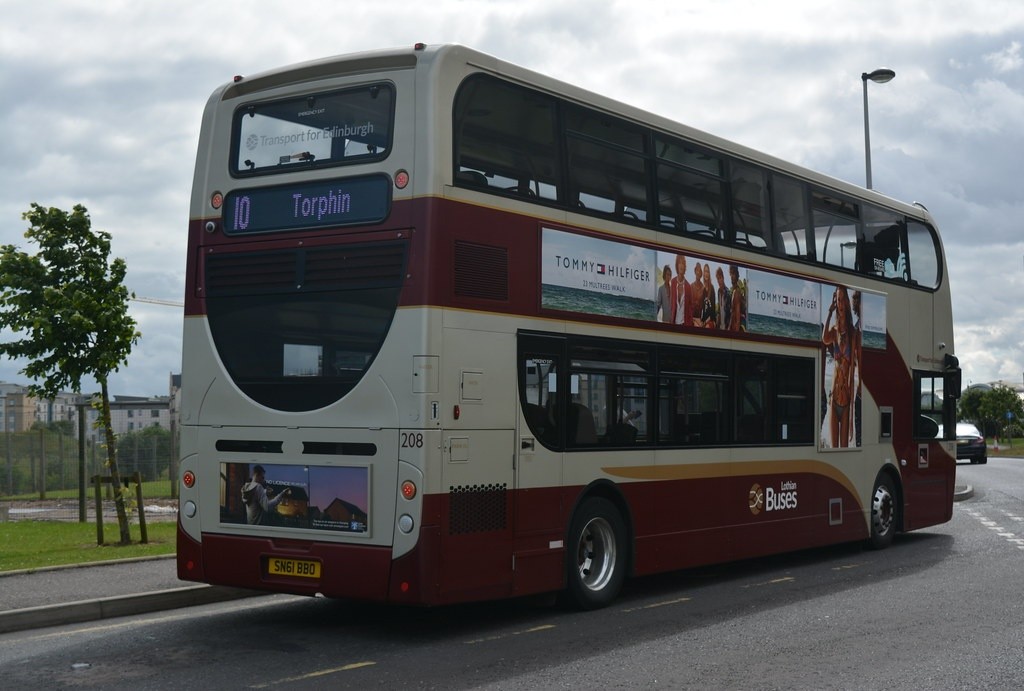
528,403,640,444
453,171,754,251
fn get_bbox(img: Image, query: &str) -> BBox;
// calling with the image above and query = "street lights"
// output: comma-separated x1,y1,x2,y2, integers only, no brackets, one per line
861,67,896,190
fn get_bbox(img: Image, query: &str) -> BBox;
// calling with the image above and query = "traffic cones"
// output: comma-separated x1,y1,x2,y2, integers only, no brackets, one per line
993,437,998,451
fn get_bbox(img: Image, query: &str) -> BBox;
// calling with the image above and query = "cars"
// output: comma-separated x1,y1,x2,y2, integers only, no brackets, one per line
937,423,987,465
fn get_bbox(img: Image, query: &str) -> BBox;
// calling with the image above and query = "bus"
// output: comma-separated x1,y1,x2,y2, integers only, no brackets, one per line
175,42,962,613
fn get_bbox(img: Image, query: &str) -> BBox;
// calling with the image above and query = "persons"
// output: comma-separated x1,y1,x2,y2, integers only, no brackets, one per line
601,391,640,427
657,254,746,332
822,285,862,448
242,466,289,525
